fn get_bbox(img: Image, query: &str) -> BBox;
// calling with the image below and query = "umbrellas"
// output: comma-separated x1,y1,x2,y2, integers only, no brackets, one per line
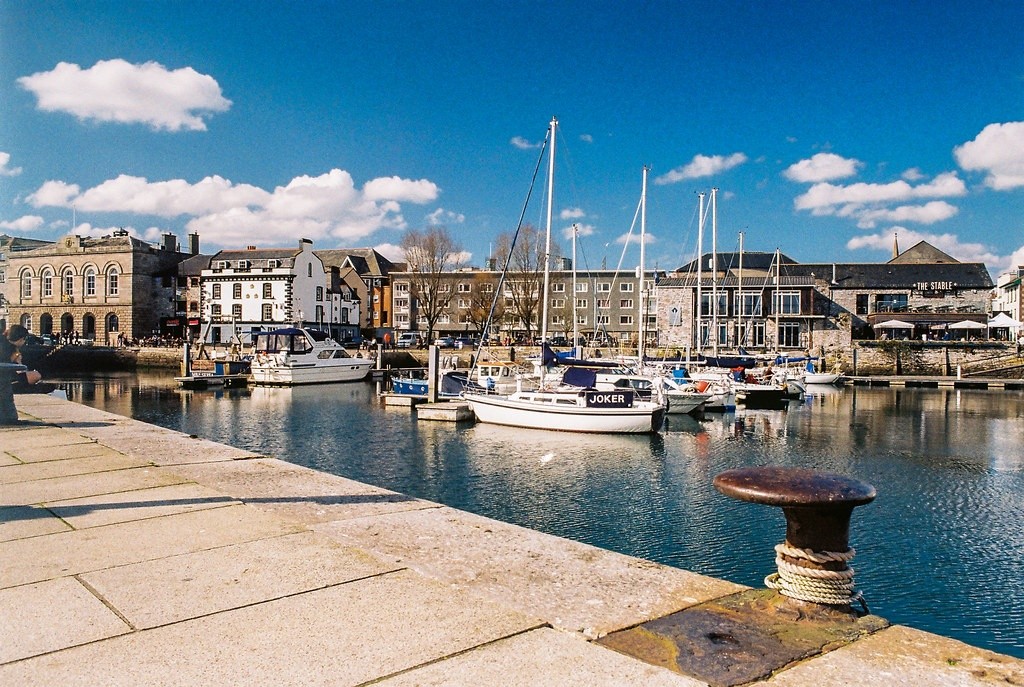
931,325,945,329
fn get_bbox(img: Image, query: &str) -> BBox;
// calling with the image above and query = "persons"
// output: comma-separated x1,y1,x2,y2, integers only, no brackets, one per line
49,330,79,345
118,332,183,348
361,332,396,351
502,334,536,347
0,325,41,390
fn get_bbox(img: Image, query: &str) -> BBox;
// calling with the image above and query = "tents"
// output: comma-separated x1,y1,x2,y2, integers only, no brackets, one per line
873,319,915,340
987,312,1023,342
948,319,987,340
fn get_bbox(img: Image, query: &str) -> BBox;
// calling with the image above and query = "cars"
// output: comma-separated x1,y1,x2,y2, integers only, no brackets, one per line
60,334,95,346
490,338,500,345
436,335,472,348
537,335,568,346
41,333,52,342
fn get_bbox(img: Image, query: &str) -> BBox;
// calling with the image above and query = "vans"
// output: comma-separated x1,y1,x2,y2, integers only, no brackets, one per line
395,331,421,347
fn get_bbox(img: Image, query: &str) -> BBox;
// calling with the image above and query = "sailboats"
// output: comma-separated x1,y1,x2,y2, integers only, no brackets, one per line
390,115,845,432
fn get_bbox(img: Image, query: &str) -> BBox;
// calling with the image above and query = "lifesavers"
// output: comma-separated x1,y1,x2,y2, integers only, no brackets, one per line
748,373,760,384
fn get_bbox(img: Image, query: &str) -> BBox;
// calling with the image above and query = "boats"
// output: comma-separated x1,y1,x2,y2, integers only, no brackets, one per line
247,328,376,384
